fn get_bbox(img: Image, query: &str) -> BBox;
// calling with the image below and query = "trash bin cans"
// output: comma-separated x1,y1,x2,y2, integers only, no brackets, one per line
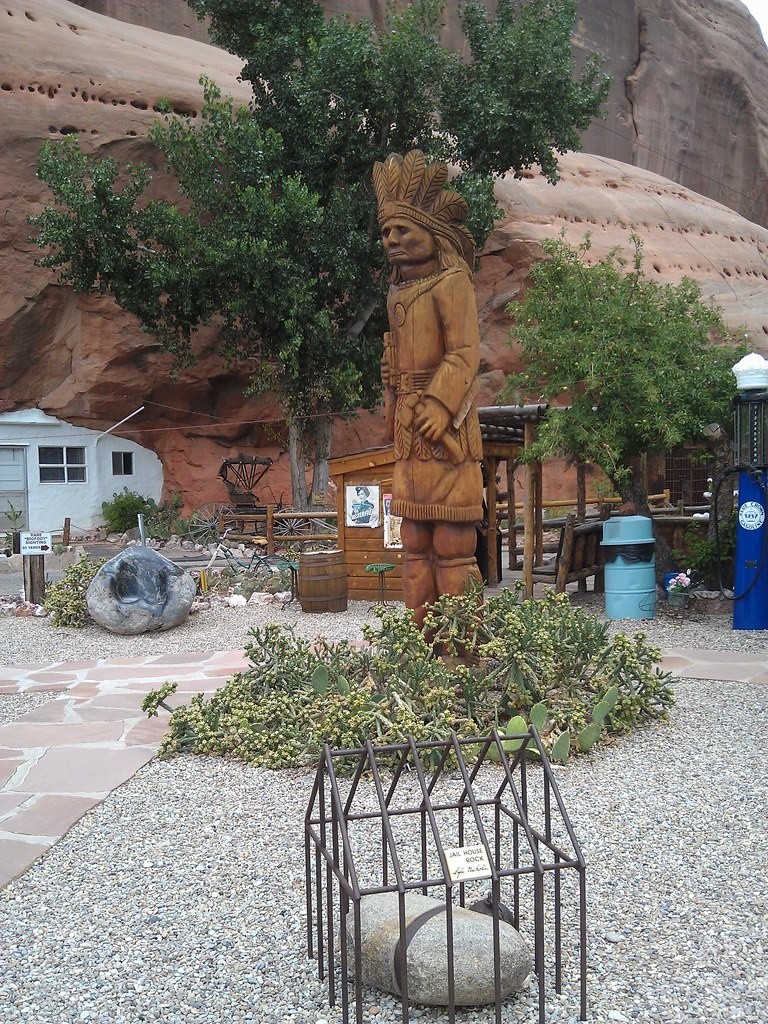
600,515,657,621
475,519,502,585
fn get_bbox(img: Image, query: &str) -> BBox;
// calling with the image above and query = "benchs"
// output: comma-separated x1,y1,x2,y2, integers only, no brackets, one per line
522,502,613,601
225,479,257,505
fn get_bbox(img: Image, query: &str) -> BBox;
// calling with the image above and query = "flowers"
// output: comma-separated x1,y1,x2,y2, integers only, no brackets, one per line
668,568,704,594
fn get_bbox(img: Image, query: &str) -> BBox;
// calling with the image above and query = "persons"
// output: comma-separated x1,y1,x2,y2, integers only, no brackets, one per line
351,487,374,525
371,148,483,665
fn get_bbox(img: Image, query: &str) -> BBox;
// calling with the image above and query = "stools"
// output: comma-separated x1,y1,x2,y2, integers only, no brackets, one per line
365,562,397,612
277,561,301,611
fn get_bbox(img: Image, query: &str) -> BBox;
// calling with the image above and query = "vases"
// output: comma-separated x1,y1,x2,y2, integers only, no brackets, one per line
668,592,689,608
